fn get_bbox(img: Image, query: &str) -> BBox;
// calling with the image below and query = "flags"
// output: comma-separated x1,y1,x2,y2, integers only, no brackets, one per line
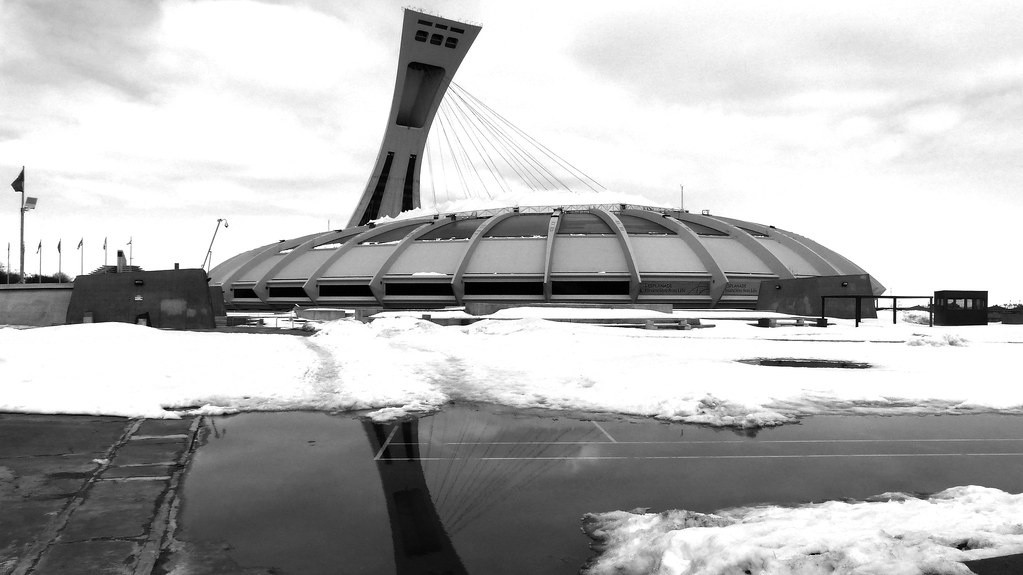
103,238,106,249
11,170,24,192
77,240,83,249
126,240,132,245
57,241,61,253
36,242,41,253
